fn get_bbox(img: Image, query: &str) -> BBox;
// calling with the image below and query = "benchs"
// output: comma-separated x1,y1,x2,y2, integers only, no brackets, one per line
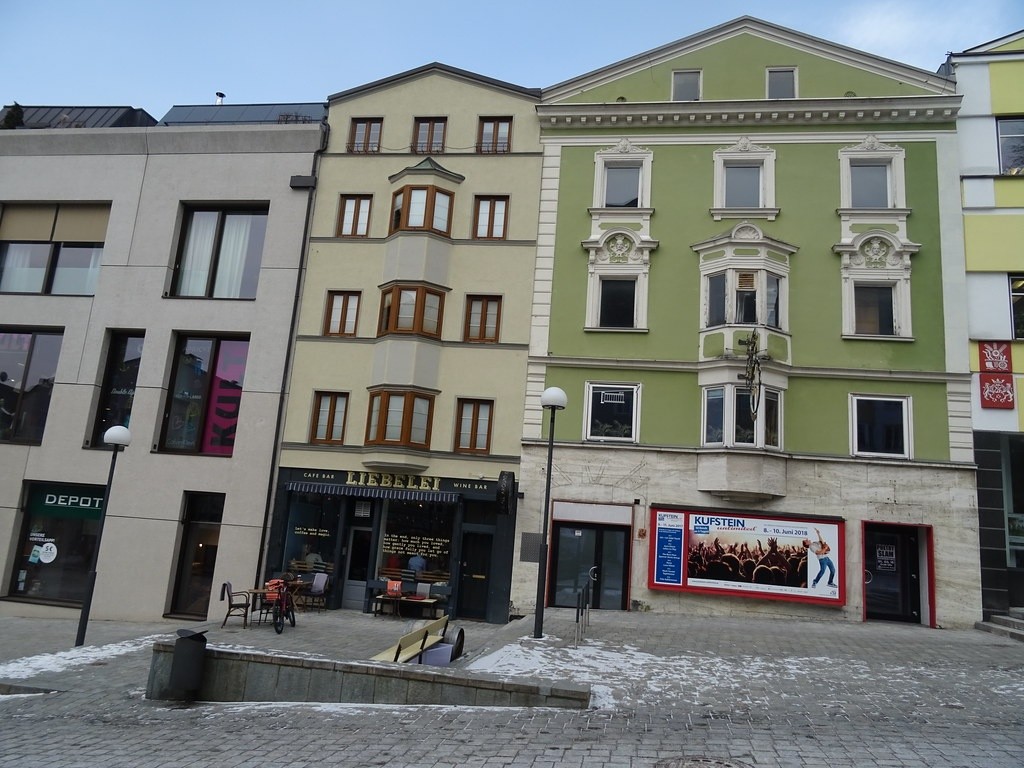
365,579,452,620
369,615,450,664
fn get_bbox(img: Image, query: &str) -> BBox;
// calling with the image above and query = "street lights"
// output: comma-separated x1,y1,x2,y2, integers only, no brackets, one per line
534,386,568,638
75,424,131,647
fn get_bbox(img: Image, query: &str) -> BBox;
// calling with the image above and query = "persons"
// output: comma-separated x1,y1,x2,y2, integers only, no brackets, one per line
803,529,837,588
387,550,427,571
305,545,323,562
688,527,808,588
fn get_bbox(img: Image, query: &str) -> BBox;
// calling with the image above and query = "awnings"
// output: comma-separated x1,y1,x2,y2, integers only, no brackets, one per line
285,480,461,505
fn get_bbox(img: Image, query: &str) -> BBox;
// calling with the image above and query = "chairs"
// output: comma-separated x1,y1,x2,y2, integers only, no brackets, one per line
303,575,337,612
221,583,251,629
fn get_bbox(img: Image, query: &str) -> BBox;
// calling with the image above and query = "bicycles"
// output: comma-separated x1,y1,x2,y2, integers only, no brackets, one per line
268,577,298,633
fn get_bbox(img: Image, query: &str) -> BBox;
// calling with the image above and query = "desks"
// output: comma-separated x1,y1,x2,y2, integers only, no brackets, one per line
249,589,284,626
286,581,310,613
375,595,437,620
